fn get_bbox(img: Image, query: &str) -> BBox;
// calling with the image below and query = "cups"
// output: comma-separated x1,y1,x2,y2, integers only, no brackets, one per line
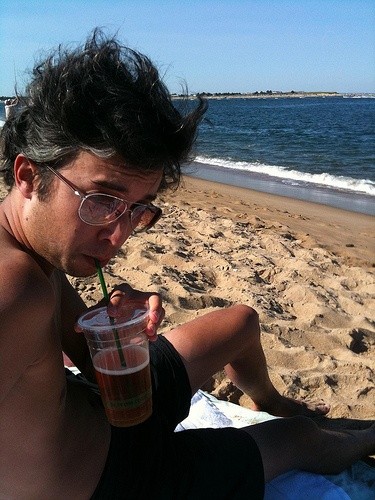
79,305,152,428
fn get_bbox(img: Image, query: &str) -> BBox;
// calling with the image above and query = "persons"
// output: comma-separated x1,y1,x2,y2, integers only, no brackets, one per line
0,28,375,500
4,97,20,121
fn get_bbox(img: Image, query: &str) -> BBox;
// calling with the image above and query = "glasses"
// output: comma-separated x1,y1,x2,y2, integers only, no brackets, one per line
44,163,163,233
